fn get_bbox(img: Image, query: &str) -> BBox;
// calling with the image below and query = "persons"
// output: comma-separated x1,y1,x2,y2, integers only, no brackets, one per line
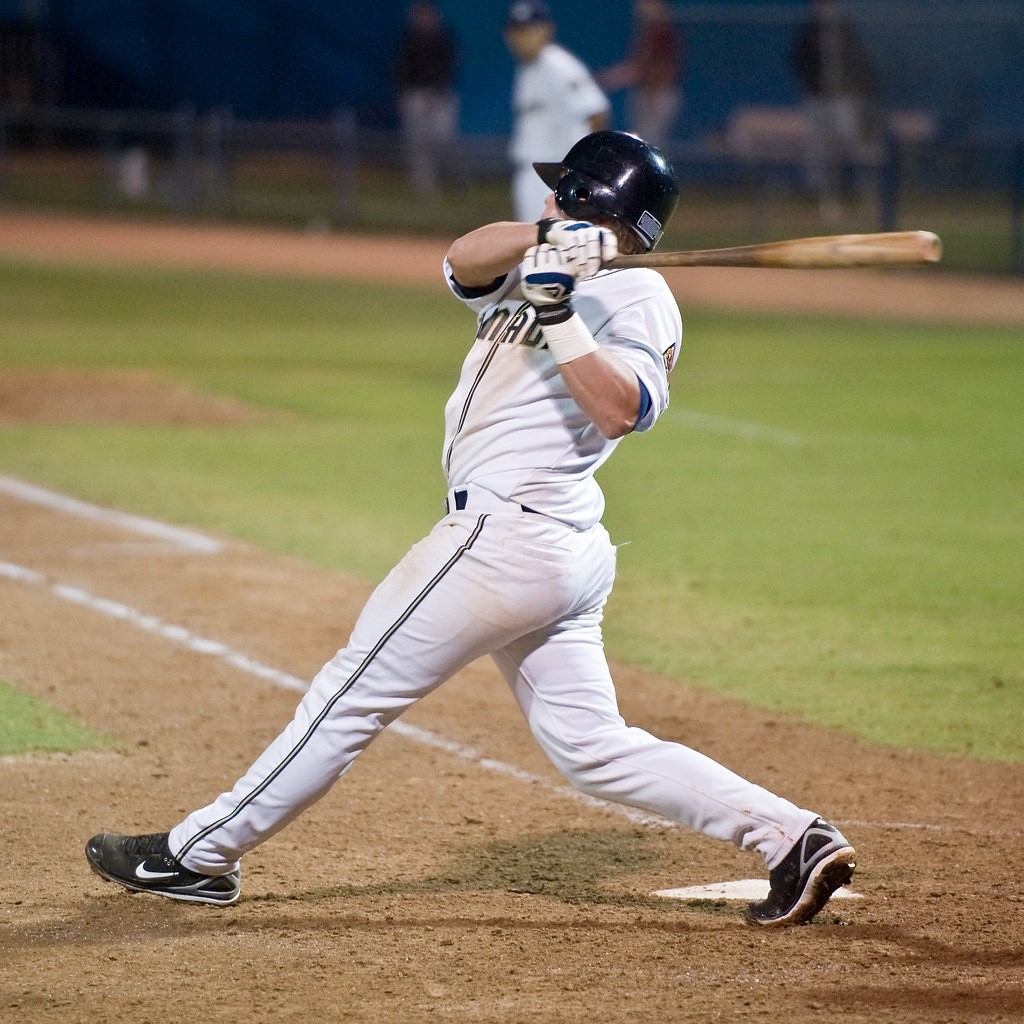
795,1,843,99
86,126,862,923
501,5,610,224
397,6,461,190
602,0,677,141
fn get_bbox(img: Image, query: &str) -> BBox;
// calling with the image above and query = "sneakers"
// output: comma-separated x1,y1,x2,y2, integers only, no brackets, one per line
751,818,857,926
84,831,240,906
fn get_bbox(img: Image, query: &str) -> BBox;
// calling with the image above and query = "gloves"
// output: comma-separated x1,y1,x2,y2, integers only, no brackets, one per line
520,242,576,306
545,220,618,277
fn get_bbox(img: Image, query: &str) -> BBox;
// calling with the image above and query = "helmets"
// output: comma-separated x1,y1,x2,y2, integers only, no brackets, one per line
532,130,680,254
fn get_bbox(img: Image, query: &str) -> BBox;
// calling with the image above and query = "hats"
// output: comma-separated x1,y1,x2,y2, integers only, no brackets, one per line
503,0,553,25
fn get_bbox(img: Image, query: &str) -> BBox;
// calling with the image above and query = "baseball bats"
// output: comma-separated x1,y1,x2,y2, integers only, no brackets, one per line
600,229,943,272
